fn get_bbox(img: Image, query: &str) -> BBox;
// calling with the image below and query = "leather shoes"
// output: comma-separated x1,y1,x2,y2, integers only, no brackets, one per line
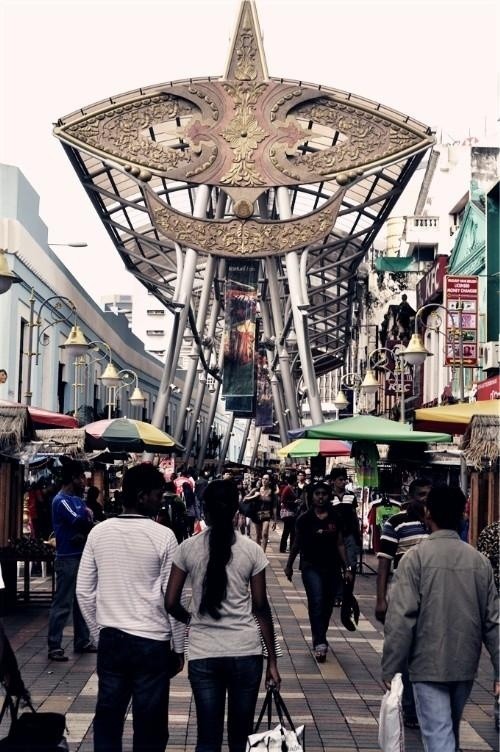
48,652,69,661
74,643,98,652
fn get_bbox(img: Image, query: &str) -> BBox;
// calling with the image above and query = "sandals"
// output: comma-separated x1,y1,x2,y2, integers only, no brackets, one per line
315,644,327,662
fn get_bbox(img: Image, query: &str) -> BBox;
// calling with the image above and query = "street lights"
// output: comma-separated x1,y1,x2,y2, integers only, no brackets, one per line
22,286,89,408
398,289,467,499
332,374,366,415
360,341,407,424
107,367,144,421
73,339,124,417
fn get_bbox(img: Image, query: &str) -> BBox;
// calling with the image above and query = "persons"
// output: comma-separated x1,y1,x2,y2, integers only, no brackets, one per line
278,467,500,750
1,462,281,752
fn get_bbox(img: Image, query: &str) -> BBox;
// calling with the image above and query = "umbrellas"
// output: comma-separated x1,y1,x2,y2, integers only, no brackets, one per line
81,415,187,474
277,399,499,575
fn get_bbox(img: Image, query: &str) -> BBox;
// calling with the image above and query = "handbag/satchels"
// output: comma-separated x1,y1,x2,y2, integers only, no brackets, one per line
378,673,406,752
238,498,257,517
340,578,360,631
192,518,208,537
0,690,69,752
244,686,306,752
